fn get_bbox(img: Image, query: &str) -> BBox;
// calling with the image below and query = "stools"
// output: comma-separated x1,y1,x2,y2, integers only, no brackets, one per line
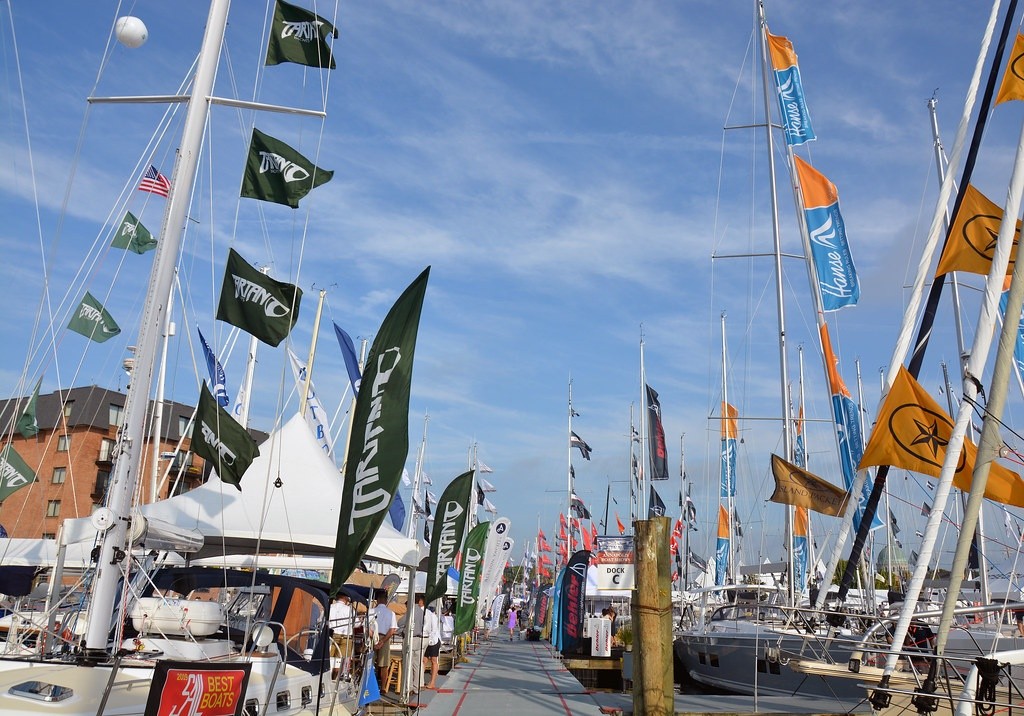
385,658,402,693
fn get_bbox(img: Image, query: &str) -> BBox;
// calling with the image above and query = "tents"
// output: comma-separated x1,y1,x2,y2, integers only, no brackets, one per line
0,413,455,677
678,555,831,602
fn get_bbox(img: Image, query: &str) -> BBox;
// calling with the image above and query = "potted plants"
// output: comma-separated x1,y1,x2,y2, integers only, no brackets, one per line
615,626,633,652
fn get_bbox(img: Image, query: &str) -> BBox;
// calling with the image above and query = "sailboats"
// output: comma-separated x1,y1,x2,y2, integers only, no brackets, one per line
617,0,1023,699
1,0,454,716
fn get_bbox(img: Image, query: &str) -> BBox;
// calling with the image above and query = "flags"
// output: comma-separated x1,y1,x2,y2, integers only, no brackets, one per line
934,185,1024,277
0,444,39,505
189,379,261,493
110,211,158,255
215,247,304,348
764,35,887,533
264,0,339,70
139,164,170,198
537,466,626,581
571,431,592,462
17,379,42,439
239,126,335,209
993,34,1024,105
857,366,1024,508
766,451,852,519
649,487,697,581
66,290,121,343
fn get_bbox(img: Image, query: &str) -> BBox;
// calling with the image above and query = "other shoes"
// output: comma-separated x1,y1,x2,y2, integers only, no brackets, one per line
510,637,513,641
381,690,386,693
426,685,435,689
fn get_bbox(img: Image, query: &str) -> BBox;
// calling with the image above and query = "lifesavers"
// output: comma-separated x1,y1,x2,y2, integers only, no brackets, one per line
42,622,74,649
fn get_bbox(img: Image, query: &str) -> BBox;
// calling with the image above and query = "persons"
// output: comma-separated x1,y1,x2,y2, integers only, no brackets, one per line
1015,611,1024,637
508,607,523,640
805,617,819,635
886,612,936,663
601,607,619,647
482,610,492,640
308,591,441,689
859,611,886,634
